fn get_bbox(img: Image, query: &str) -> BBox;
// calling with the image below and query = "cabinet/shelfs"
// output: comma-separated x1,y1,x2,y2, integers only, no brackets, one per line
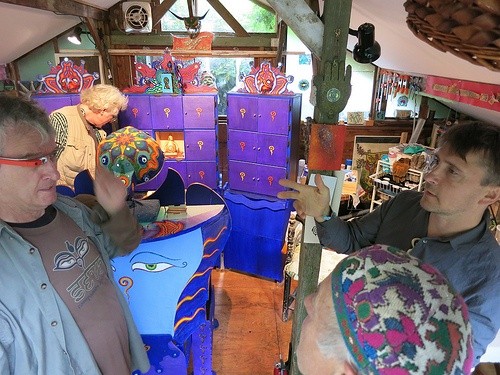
227,91,301,197
369,159,432,215
30,93,83,115
119,93,220,190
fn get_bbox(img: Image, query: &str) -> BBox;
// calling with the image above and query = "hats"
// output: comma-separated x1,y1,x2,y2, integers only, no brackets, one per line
96,126,169,198
332,245,473,375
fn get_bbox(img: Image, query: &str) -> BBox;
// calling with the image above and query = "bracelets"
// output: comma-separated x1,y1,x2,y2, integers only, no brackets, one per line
320,206,336,220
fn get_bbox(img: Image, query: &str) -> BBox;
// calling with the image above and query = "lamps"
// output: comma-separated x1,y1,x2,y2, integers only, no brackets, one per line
349,24,381,63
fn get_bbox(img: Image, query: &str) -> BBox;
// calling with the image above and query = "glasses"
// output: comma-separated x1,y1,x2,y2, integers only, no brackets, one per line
0,152,59,167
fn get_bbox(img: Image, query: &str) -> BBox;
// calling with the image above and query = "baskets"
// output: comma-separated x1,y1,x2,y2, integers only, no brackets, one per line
404,0,500,72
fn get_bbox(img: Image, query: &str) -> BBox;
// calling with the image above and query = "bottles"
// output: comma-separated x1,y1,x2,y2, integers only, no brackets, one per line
297,159,309,185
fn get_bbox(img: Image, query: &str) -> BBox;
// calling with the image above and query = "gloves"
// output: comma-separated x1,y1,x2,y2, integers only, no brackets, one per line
393,158,411,183
313,60,353,115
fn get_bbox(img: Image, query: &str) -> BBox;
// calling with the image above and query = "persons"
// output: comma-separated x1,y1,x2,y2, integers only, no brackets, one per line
276,121,500,375
0,94,152,375
47,83,128,192
